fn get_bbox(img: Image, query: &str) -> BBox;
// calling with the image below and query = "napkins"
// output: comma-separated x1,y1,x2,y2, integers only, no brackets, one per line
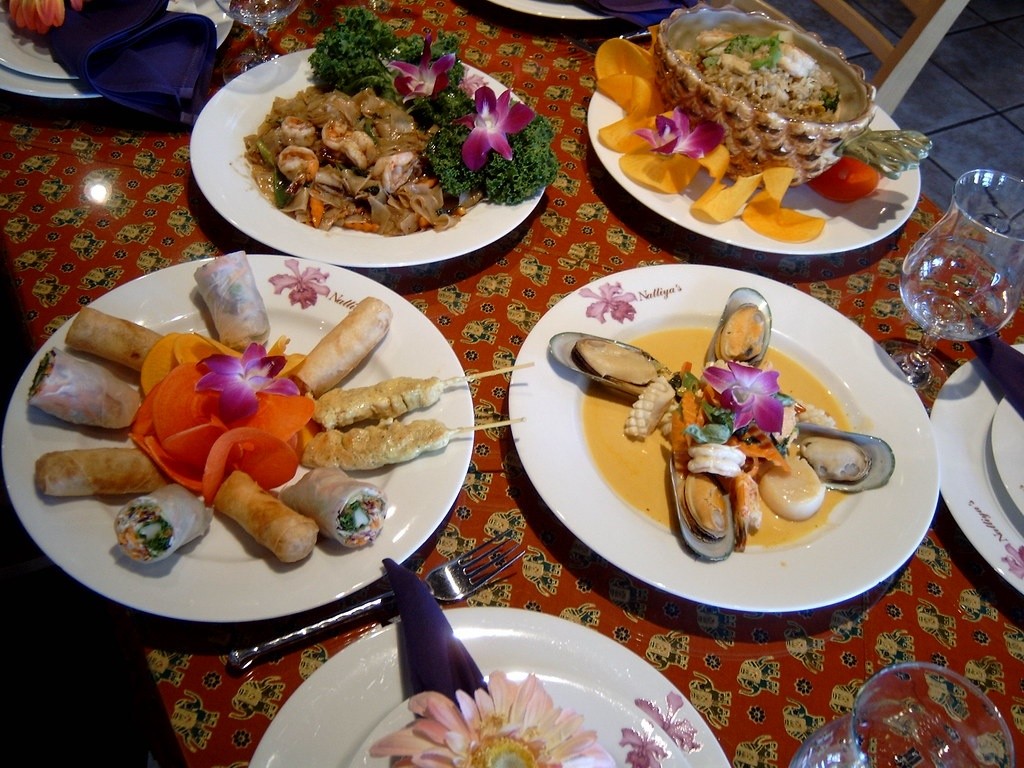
382,558,490,719
48,0,217,125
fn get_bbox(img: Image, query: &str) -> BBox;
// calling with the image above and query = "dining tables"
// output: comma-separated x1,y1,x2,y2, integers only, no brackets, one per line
0,0,1024,768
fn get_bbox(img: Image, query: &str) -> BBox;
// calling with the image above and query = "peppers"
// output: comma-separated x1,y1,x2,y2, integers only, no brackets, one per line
676,371,701,397
255,141,288,208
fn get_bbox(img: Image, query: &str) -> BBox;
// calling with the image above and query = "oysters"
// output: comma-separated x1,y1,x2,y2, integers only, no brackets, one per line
549,287,896,562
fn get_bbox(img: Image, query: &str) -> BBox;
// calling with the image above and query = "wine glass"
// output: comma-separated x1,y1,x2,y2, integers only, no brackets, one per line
215,0,301,86
787,661,1015,768
880,168,1024,415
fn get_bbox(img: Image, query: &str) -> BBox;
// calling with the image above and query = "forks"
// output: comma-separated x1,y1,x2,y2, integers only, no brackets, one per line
226,528,527,675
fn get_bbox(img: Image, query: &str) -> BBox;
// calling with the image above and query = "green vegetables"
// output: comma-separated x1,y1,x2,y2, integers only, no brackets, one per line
704,34,843,112
309,9,559,206
684,394,797,455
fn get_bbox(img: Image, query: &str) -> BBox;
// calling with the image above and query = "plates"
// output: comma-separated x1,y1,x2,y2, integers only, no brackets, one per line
189,47,547,268
586,91,922,257
488,0,615,21
0,0,234,100
929,343,1024,598
508,262,943,614
0,254,476,625
247,606,732,768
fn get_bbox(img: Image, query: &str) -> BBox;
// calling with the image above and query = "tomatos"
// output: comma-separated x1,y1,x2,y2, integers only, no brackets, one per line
812,156,880,202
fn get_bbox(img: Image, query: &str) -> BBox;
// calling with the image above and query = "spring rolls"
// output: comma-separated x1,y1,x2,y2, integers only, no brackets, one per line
28,251,390,562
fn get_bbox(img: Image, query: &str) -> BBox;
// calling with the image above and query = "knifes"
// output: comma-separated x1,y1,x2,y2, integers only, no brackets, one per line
967,336,1024,418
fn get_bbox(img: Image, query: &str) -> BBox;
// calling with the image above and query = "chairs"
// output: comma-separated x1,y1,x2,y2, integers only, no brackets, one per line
711,0,971,118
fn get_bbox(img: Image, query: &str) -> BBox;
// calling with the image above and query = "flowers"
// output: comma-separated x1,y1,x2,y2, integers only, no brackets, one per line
7,0,83,34
196,341,301,423
453,84,536,170
702,361,784,433
368,671,615,768
633,109,723,161
387,35,457,105
